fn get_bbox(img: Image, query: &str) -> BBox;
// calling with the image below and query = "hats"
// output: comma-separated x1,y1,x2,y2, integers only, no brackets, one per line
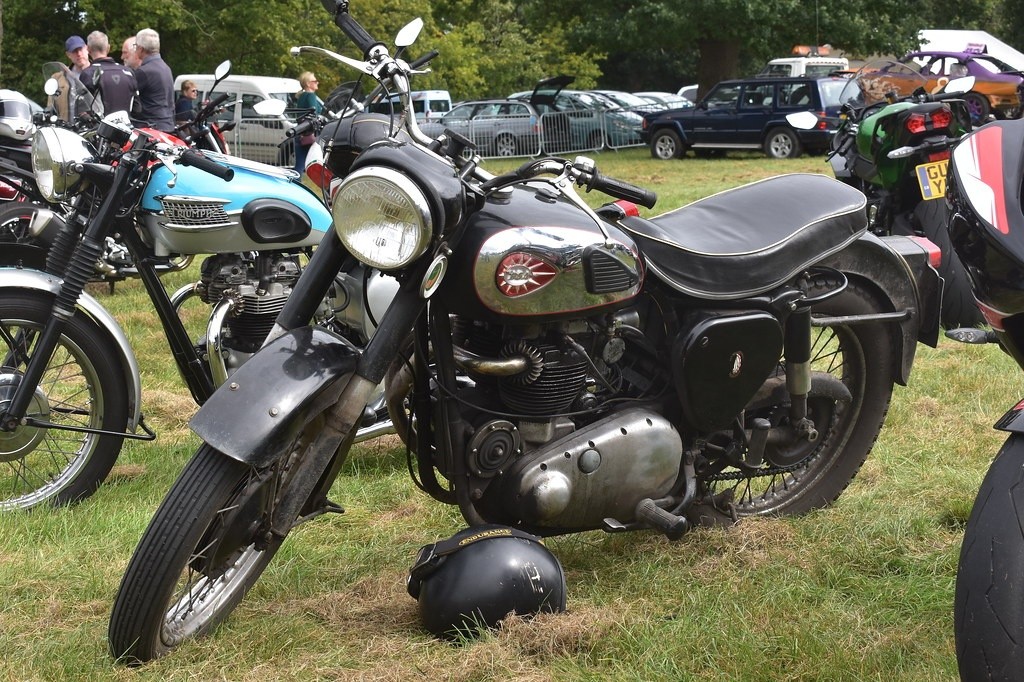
64,36,87,53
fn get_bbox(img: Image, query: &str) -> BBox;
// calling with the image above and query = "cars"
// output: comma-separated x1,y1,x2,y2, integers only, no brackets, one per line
852,43,1024,127
676,83,743,110
415,75,578,159
584,86,662,144
632,91,697,111
482,89,646,146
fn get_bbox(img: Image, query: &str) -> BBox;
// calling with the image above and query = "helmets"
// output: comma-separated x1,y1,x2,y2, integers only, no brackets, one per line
0,89,32,142
409,527,566,645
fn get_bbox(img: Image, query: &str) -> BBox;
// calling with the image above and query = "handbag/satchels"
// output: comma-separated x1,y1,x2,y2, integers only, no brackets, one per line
299,119,315,145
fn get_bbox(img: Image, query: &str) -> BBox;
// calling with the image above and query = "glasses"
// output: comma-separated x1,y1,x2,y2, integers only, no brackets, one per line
191,90,200,94
310,80,318,83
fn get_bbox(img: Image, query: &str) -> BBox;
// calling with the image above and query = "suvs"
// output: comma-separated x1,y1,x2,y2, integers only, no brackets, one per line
641,73,865,160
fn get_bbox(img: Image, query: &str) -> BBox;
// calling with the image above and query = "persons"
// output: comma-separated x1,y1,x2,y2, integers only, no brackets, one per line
292,71,330,202
43,28,199,134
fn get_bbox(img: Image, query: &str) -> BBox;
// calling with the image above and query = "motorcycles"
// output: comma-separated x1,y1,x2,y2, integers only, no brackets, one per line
824,56,998,333
942,112,1024,682
109,0,947,668
0,0,641,513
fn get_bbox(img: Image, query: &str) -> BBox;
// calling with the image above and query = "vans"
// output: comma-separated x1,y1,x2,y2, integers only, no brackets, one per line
173,72,330,168
752,44,848,109
363,90,452,127
